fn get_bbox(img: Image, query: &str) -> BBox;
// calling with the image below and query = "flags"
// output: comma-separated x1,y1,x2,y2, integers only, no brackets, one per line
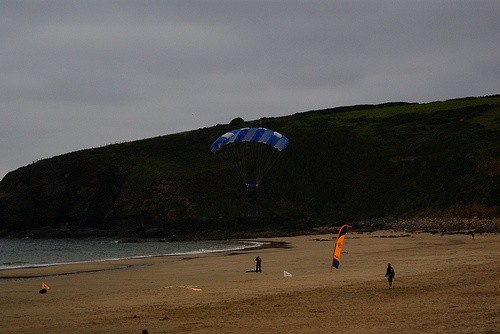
332,224,348,269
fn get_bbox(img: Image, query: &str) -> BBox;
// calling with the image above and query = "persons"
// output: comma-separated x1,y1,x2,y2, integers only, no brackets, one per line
385,263,395,289
255,255,262,273
